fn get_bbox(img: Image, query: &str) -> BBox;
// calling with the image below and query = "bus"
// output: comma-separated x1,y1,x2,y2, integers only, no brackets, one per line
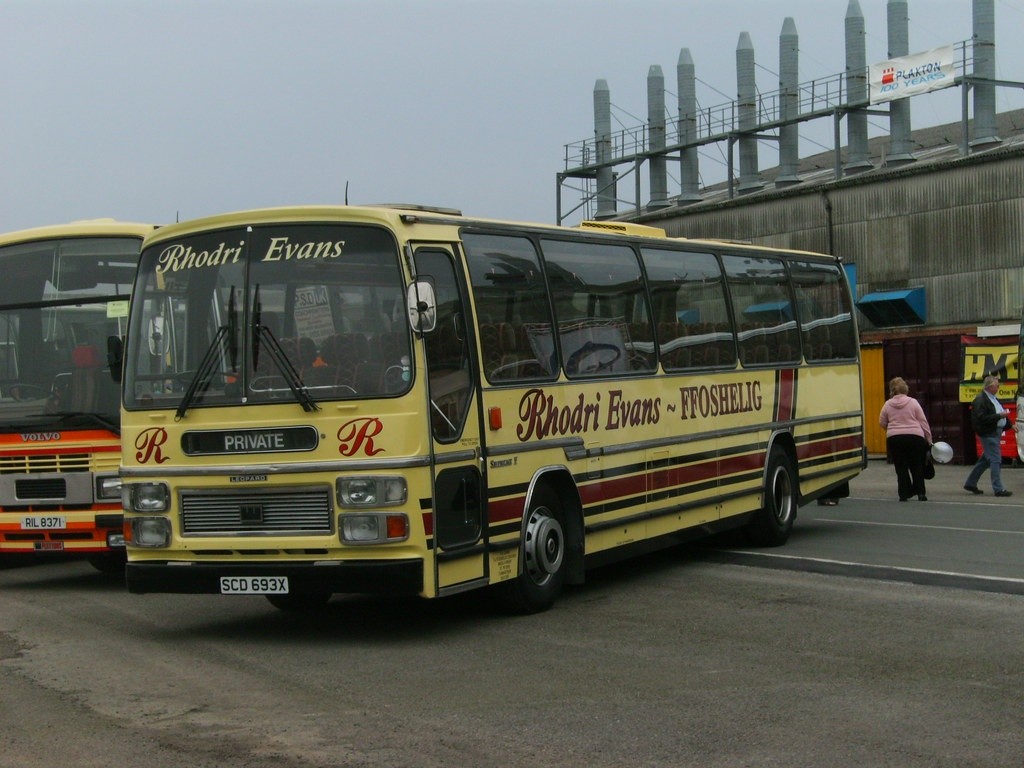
114,201,867,616
0,218,356,579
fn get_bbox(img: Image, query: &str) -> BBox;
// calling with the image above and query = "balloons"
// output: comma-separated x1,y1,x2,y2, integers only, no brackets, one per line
931,441,953,463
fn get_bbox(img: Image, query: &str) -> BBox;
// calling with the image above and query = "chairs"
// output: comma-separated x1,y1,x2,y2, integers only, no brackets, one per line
226,321,832,437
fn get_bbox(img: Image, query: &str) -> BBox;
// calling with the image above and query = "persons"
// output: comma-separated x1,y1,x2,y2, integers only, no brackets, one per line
964,374,1019,497
817,480,851,507
879,377,933,501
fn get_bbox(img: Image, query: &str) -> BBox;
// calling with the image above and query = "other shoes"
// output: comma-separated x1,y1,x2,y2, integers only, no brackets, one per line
899,498,907,501
994,489,1012,497
818,499,837,506
964,485,983,495
918,495,927,501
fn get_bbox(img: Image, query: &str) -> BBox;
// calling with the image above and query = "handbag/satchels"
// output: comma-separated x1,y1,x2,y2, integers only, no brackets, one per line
923,445,935,479
